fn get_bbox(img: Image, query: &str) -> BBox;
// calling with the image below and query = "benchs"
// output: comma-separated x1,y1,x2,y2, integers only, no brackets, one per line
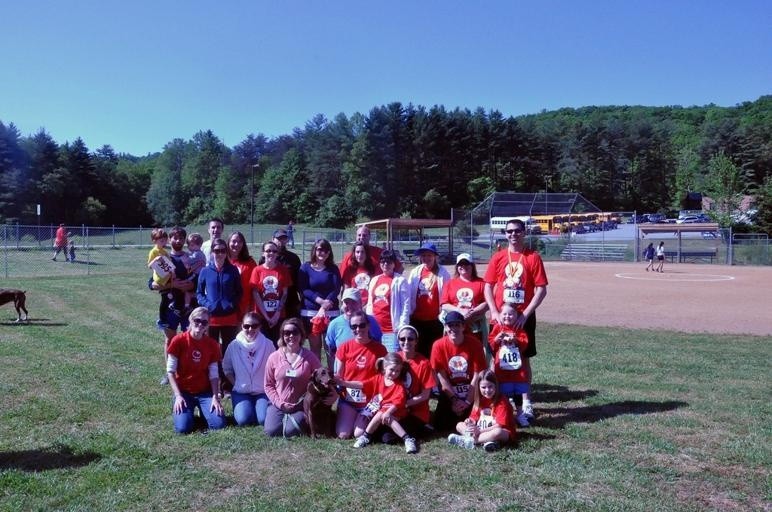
402,249,448,262
643,247,719,265
560,244,629,261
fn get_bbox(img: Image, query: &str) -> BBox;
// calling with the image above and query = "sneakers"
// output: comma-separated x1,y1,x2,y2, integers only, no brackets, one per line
404,437,416,455
351,436,368,449
166,300,194,320
517,403,536,427
483,441,501,452
159,375,170,386
448,432,473,448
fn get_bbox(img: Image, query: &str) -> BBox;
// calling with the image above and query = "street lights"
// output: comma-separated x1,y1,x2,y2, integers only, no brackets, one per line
248,162,263,248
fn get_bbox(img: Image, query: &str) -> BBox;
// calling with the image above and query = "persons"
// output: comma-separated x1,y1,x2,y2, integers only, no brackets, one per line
439,253,486,349
286,221,295,249
177,235,206,313
224,231,257,323
448,368,518,454
428,312,488,434
325,288,383,379
297,239,341,379
201,218,224,269
341,241,376,310
394,325,436,440
147,228,183,315
249,241,290,350
406,244,450,359
258,228,301,316
67,240,78,262
48,221,71,265
263,316,339,437
196,239,243,400
364,251,411,352
341,225,405,290
487,300,539,430
333,352,419,454
482,218,549,418
333,311,390,438
165,306,226,435
655,240,667,272
222,310,277,428
147,226,206,384
641,242,656,271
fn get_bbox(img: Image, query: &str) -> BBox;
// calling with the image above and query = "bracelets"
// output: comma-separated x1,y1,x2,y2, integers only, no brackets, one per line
464,399,472,406
449,394,459,402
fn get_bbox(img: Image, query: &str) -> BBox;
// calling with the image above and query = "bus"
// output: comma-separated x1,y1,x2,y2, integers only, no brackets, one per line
489,212,622,237
614,211,634,223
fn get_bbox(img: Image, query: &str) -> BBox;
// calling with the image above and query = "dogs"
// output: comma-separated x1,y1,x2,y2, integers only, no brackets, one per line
0,288,28,322
301,366,334,441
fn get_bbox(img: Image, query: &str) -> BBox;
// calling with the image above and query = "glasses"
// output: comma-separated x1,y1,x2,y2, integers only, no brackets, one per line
191,317,209,328
399,335,416,343
213,247,228,255
506,228,525,235
283,329,302,336
243,322,260,330
349,322,369,330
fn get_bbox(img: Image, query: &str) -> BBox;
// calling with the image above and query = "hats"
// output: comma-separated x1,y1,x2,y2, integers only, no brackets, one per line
455,253,475,266
444,311,465,326
414,241,439,258
341,288,361,304
272,229,289,239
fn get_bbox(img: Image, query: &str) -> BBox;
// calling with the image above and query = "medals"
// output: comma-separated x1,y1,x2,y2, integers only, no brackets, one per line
507,280,514,287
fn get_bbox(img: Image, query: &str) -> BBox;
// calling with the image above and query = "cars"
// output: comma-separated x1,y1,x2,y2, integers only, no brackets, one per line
299,228,434,240
627,210,759,230
